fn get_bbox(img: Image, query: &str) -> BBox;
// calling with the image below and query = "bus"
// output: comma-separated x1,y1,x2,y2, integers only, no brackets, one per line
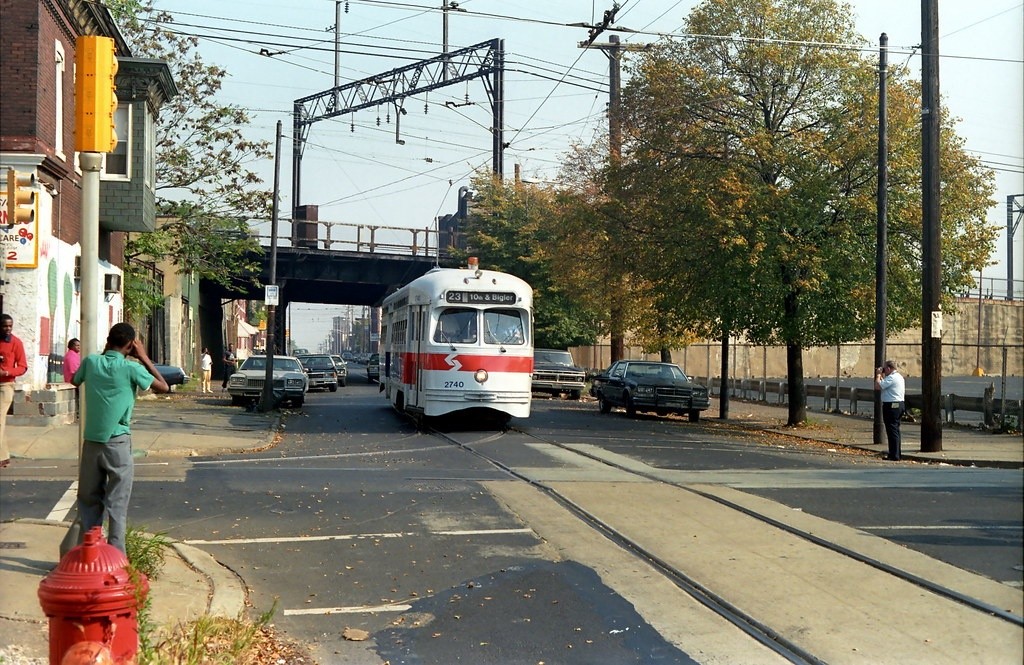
376,258,534,430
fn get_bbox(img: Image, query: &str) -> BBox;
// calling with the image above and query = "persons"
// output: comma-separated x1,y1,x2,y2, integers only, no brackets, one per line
71,322,169,557
201,347,213,394
439,310,460,336
63,338,80,419
876,360,905,461
491,318,520,339
222,343,237,391
0,314,27,467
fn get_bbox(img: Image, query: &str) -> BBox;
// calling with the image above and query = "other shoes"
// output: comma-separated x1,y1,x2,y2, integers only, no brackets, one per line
882,456,888,459
222,388,227,392
202,391,205,394
0,459,11,467
206,390,213,393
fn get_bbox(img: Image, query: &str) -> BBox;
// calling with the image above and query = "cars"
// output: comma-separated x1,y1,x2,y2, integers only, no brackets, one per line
531,348,585,400
589,360,711,423
227,353,310,408
292,356,348,390
367,354,382,383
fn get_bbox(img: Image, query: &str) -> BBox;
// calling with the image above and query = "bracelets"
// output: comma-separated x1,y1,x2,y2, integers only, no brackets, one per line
6,371,8,377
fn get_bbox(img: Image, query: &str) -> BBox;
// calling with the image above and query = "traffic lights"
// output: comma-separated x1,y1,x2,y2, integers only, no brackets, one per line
6,170,38,229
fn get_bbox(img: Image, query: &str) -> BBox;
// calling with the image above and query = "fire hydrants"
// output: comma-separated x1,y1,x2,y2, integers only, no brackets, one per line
37,522,150,665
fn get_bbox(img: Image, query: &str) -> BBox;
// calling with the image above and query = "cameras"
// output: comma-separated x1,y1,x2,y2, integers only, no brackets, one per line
129,346,135,355
882,368,885,373
